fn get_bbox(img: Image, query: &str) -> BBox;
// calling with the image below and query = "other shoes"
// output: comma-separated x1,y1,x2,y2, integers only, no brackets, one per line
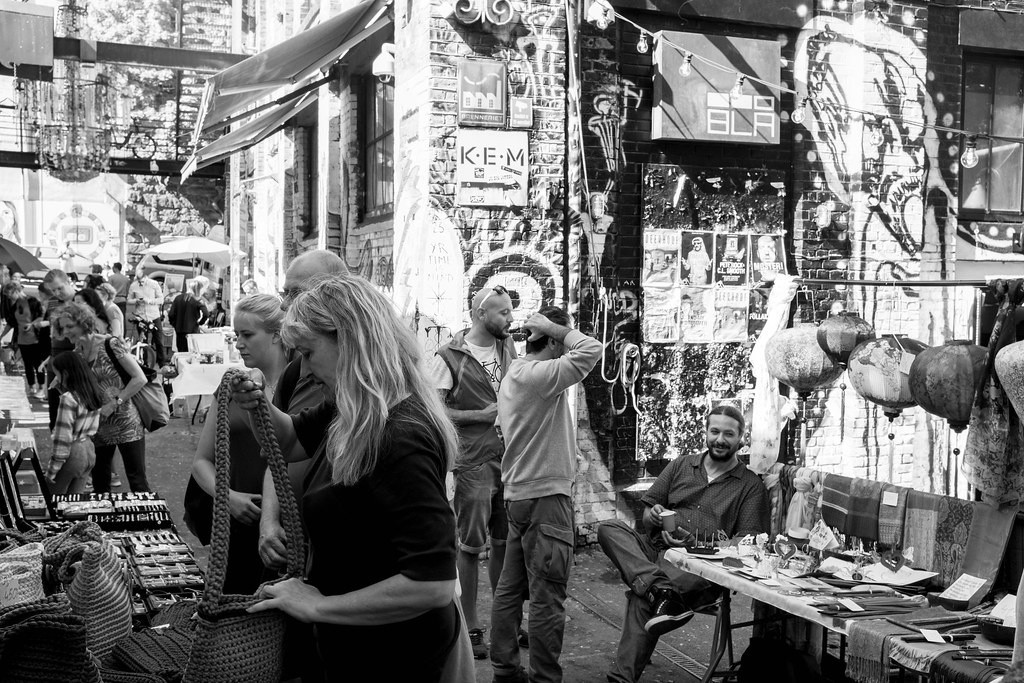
493,667,529,683
110,472,122,486
26,387,45,399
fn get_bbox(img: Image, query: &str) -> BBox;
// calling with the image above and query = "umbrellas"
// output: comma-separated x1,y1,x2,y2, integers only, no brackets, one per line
137,236,246,279
0,237,50,276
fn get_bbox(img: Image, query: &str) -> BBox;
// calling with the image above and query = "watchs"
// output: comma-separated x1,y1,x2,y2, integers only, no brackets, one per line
115,396,123,405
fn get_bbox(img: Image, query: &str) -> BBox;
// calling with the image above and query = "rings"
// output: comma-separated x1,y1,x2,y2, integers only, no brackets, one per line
263,554,268,559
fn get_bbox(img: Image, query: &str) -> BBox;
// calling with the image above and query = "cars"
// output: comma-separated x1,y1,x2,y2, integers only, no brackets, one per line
15,245,66,282
134,249,220,285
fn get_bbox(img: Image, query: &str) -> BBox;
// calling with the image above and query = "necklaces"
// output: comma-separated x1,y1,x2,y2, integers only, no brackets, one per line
86,336,94,359
266,383,277,394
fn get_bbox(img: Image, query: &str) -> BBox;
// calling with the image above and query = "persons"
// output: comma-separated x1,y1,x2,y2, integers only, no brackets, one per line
682,236,712,284
490,307,604,683
753,236,784,281
426,286,531,660
598,406,770,683
0,262,225,497
241,278,258,295
191,250,476,683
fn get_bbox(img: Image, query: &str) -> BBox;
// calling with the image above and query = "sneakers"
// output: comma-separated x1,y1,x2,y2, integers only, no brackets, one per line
516,628,529,647
469,628,488,658
644,585,695,634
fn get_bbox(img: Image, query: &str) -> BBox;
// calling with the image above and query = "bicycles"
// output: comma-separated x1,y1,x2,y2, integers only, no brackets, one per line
86,112,157,160
122,312,159,370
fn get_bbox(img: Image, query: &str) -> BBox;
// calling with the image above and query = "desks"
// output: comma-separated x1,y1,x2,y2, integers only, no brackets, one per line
664,546,1013,682
168,352,244,425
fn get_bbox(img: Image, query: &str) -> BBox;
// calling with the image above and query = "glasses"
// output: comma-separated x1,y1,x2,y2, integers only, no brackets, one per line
480,285,509,307
278,290,303,302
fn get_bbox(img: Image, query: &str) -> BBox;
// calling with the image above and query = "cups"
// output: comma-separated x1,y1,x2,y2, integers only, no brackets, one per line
659,511,675,532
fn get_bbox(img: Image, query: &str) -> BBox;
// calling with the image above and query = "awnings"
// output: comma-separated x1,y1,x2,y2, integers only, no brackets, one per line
180,0,396,185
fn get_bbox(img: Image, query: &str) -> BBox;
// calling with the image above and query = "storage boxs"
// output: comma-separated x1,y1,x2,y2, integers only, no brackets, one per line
186,333,225,353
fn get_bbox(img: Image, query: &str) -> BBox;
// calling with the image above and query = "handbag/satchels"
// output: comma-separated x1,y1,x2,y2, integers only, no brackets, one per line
182,474,214,546
180,368,305,683
105,336,170,432
43,520,133,662
0,523,108,683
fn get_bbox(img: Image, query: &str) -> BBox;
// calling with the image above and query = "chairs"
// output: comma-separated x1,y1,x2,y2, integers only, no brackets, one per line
647,591,738,683
